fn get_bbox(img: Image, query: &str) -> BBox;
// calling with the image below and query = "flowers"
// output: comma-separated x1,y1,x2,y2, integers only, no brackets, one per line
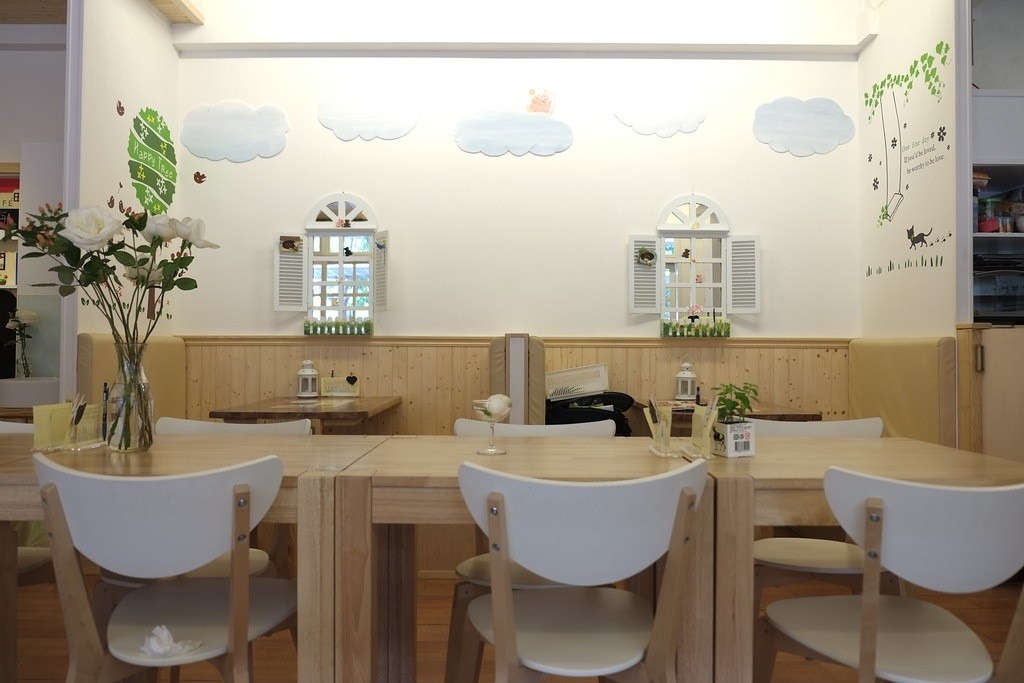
1,202,220,449
2,304,40,375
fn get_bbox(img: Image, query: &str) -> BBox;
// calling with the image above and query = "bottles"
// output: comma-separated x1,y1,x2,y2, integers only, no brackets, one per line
979,197,1002,232
973,197,979,232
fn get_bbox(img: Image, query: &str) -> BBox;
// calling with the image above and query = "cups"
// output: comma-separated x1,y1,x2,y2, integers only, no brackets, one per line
998,217,1012,232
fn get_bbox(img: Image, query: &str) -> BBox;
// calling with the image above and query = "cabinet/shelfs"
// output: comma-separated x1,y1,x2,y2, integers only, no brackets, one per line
971,160,1024,325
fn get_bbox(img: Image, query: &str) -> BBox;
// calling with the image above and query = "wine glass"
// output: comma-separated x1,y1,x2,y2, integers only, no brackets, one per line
471,399,512,456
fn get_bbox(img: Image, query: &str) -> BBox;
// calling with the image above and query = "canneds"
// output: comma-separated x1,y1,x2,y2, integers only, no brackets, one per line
998,217,1005,232
1005,217,1013,232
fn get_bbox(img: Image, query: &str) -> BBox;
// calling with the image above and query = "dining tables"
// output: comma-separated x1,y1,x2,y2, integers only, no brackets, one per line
652,436,1024,681
335,435,716,680
1,431,392,683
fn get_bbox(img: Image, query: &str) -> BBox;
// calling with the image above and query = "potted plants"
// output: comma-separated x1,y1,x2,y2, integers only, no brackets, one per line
709,382,759,457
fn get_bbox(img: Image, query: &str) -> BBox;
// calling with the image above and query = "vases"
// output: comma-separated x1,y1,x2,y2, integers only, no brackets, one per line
105,342,155,452
16,348,33,377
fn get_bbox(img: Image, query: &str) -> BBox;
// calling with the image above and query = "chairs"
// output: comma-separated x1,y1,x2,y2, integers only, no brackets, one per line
725,415,888,682
455,458,709,681
91,415,311,682
33,451,298,682
441,417,616,683
752,466,1024,682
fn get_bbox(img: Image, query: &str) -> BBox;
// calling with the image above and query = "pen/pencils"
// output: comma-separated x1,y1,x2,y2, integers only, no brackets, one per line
649,401,659,423
696,387,701,405
101,382,109,441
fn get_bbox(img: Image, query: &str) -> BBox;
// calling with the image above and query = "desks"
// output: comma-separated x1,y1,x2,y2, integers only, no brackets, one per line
209,395,403,435
648,397,822,437
